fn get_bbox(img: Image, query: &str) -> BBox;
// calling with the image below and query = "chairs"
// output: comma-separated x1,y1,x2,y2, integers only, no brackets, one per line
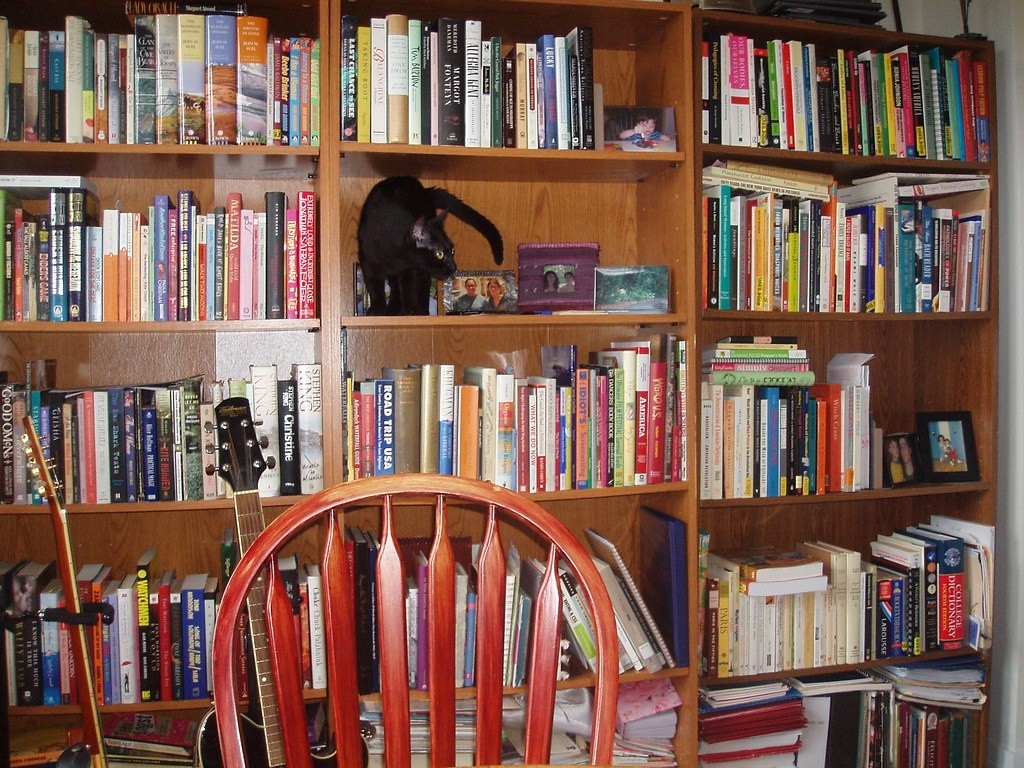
208,474,620,768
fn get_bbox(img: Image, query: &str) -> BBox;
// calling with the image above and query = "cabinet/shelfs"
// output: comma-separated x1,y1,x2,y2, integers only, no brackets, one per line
0,0,998,768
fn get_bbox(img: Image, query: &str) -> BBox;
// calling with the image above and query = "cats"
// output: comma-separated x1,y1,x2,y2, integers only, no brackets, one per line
356,174,504,316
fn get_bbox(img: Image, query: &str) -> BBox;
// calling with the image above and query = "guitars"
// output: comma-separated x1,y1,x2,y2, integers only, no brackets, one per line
193,396,372,768
23,413,112,768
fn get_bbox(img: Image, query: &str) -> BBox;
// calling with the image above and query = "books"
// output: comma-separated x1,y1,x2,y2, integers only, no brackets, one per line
355,677,682,767
0,525,328,711
702,155,990,314
696,515,994,674
0,0,322,147
699,28,990,163
339,13,596,152
7,711,201,768
338,503,695,689
0,172,318,320
0,358,324,507
691,0,887,28
699,334,871,502
344,333,688,497
698,656,989,768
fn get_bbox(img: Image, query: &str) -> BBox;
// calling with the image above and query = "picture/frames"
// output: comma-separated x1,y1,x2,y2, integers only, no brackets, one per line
917,411,979,483
442,270,518,314
883,433,925,490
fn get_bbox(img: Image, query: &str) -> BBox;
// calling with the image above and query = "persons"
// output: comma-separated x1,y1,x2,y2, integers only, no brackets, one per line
544,270,558,292
888,434,962,488
559,272,576,292
452,279,485,311
619,111,671,142
486,279,508,310
